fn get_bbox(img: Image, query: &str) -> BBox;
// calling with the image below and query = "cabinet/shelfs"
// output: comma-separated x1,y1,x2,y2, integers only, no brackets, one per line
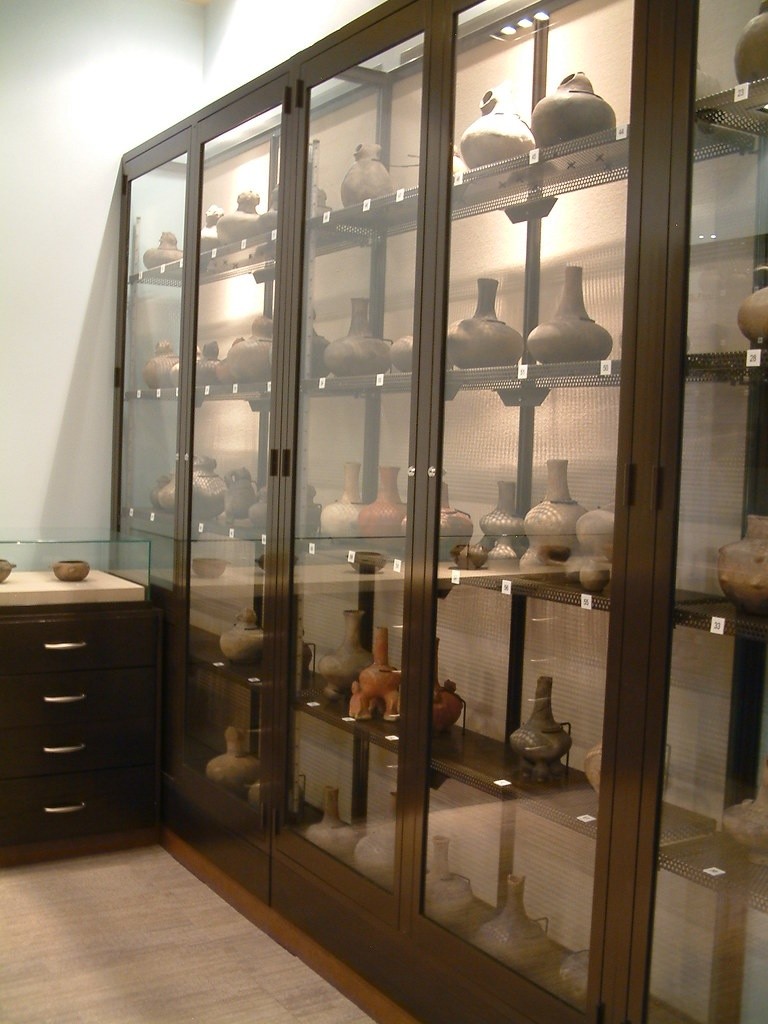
103,0,766,1024
2,602,164,868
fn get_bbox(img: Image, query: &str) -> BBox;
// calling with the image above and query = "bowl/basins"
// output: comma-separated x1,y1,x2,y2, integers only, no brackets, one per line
53,559,91,582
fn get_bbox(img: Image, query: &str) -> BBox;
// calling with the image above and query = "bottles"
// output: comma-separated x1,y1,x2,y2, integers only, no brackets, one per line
135,2,767,1007
